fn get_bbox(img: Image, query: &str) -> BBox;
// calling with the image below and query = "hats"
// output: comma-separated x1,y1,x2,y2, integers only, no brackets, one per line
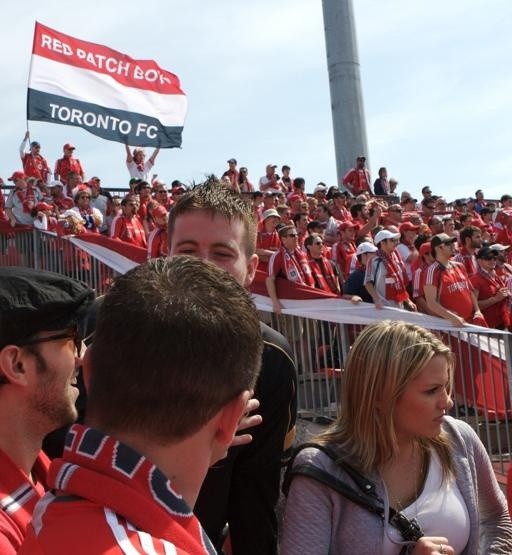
46,180,64,188
153,205,167,218
357,156,366,161
129,177,142,186
227,158,237,164
64,144,75,150
7,172,25,181
35,201,53,212
339,198,510,258
265,163,277,168
262,184,348,221
2,267,96,342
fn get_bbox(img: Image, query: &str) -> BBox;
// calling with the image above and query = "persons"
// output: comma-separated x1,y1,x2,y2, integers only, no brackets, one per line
37,172,298,554
171,186,188,211
0,263,96,554
237,164,254,193
255,155,512,416
221,157,239,192
220,175,231,192
15,256,264,554
1,130,172,290
280,318,512,555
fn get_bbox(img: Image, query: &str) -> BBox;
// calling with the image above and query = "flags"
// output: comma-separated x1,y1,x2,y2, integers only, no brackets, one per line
25,19,188,149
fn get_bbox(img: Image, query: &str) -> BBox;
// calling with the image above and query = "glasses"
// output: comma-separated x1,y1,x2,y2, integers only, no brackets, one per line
76,181,190,210
486,256,497,260
290,234,298,236
427,190,432,194
317,241,325,245
35,327,85,348
